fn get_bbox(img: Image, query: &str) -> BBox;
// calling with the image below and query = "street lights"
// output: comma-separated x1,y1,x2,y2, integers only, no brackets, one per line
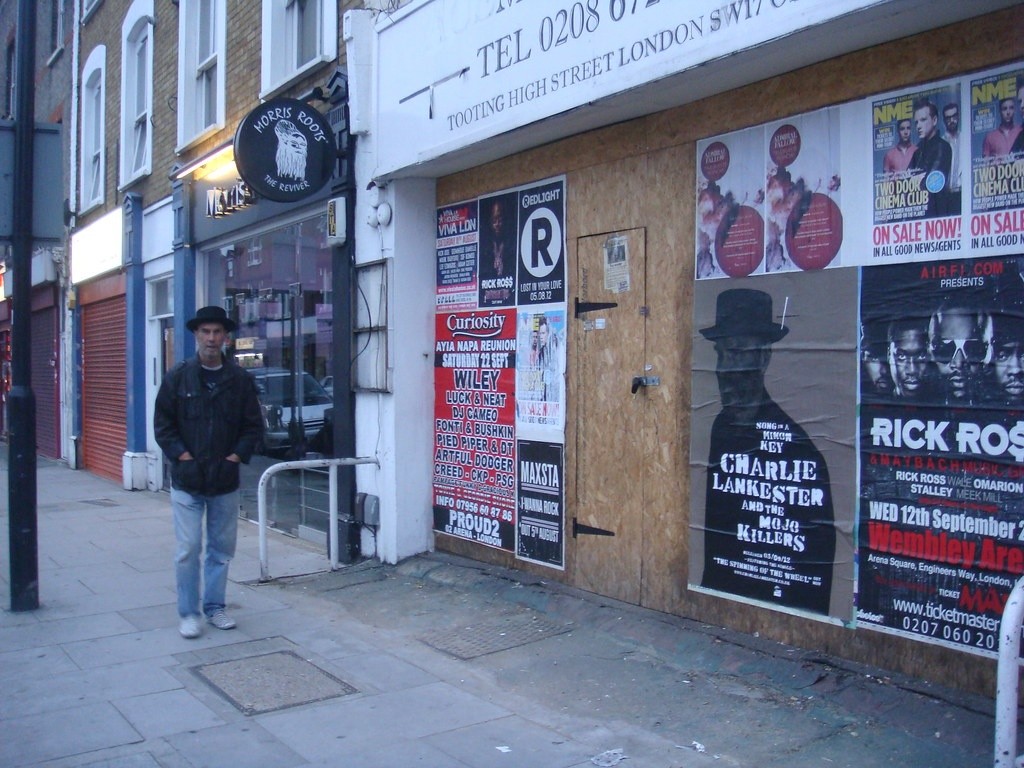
288,281,302,445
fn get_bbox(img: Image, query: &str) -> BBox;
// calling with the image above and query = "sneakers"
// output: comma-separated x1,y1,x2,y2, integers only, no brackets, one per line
206,610,236,630
179,614,200,638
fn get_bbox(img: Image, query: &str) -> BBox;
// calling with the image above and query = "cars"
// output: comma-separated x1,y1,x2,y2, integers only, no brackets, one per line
246,367,333,458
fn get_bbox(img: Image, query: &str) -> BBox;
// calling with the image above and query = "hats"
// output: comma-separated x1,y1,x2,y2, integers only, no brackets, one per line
186,307,236,334
698,289,789,343
860,326,890,349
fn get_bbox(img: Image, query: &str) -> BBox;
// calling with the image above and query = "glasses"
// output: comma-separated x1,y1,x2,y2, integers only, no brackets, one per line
945,113,959,122
931,338,989,364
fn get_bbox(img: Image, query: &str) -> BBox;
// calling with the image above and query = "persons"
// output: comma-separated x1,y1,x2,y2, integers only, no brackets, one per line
697,289,837,616
860,295,1024,404
530,317,549,401
154,306,264,640
883,76,1024,224
